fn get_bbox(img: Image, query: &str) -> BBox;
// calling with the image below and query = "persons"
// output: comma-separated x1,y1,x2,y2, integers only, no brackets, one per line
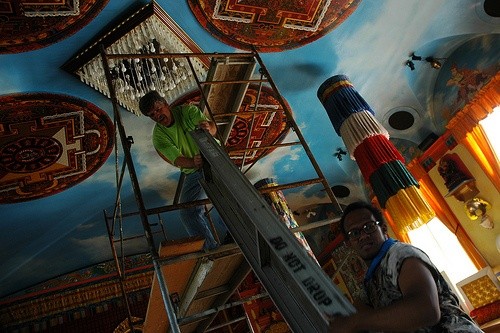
325,201,484,333
137,90,222,253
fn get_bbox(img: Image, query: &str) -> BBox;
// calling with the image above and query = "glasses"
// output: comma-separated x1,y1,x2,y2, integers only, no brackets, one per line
345,221,380,242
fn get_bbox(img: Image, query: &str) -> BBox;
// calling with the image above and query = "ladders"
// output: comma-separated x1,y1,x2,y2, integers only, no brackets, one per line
190,127,359,333
